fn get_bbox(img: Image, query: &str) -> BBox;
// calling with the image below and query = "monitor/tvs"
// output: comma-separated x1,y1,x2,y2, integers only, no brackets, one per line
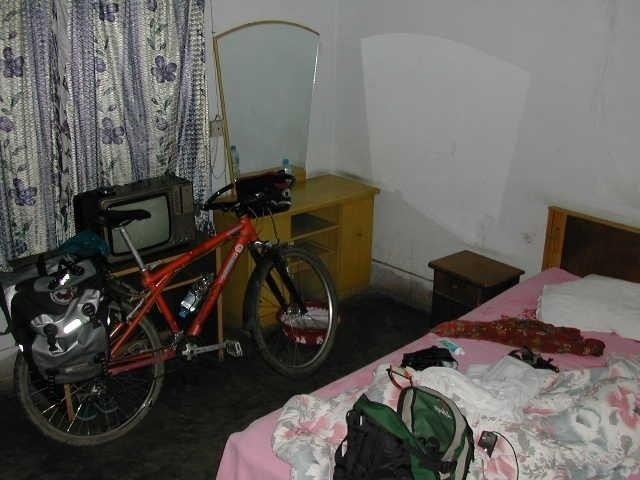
73,175,195,264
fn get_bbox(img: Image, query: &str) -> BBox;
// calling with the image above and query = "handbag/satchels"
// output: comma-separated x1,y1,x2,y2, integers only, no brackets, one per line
207,169,296,220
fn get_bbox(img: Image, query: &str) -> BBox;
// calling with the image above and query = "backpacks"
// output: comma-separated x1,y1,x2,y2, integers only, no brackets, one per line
333,385,475,480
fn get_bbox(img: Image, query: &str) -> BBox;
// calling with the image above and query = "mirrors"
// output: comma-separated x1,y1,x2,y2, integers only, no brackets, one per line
213,20,320,187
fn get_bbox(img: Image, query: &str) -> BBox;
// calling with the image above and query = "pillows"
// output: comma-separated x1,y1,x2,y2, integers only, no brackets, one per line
536,274,640,341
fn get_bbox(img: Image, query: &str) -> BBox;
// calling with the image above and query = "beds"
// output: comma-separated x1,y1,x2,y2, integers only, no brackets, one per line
216,206,640,480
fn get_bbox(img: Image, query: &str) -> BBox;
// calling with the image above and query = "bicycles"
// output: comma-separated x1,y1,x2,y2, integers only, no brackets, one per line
1,171,341,447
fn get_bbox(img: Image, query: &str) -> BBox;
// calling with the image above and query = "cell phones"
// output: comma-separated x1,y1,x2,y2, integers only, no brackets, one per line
478,430,498,456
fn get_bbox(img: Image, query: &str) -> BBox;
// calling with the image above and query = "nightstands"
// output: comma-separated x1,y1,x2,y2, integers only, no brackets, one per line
428,250,525,329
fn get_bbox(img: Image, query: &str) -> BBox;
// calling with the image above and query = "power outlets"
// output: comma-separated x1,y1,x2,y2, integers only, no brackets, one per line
211,120,224,137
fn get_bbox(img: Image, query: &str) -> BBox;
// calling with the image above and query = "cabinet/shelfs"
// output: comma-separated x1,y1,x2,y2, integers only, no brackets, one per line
60,230,223,421
214,175,380,331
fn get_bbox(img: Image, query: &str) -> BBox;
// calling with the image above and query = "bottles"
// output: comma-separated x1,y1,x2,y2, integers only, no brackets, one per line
229,143,241,183
279,158,294,209
178,273,216,320
